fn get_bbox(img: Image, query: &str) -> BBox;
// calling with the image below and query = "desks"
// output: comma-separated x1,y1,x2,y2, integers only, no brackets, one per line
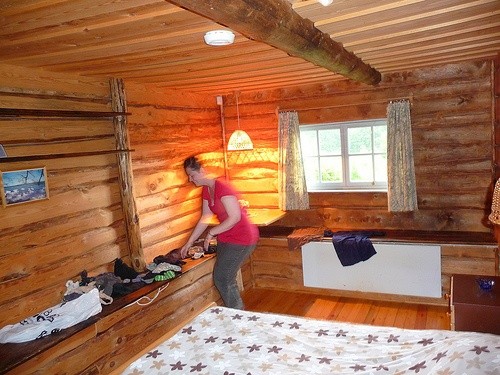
201,208,288,227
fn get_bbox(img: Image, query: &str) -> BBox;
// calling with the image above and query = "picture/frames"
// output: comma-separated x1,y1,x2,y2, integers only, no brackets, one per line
0,165,50,209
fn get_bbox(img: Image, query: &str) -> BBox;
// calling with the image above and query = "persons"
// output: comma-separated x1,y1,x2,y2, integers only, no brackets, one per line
179,155,259,311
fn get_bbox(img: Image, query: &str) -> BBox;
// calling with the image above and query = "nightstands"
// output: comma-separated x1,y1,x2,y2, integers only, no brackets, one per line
444,274,500,335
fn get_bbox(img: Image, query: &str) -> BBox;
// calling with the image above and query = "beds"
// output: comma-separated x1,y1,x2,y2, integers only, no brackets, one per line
109,302,500,375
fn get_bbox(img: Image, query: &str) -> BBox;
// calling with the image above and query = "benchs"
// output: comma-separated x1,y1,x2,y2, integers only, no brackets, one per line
249,228,500,306
0,240,252,375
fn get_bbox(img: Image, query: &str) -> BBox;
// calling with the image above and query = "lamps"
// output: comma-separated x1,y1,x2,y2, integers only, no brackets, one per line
204,30,236,46
227,93,254,150
